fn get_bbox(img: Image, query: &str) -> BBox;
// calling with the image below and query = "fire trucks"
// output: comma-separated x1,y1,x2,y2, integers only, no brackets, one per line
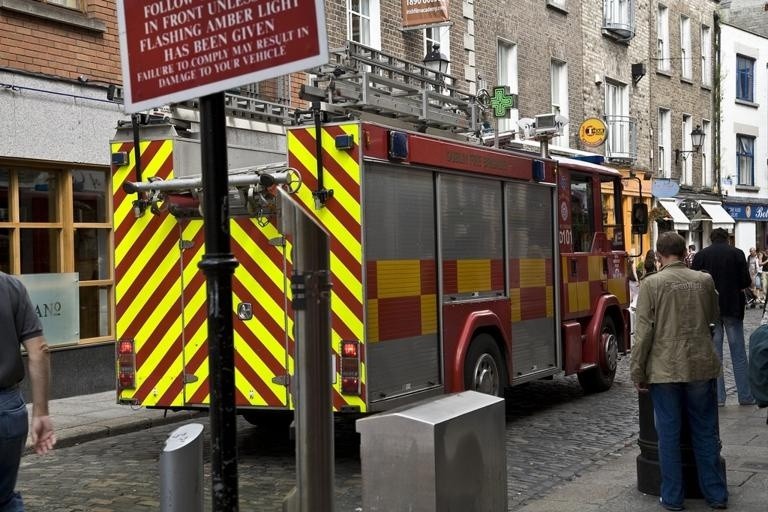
94,39,654,447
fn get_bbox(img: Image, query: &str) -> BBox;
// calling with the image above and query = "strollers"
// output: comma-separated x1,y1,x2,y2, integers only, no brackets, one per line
741,282,766,310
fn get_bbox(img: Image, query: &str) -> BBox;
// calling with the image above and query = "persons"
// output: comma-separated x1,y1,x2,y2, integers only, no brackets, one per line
1,270,57,512
626,243,768,311
568,191,596,252
629,231,729,511
686,227,755,409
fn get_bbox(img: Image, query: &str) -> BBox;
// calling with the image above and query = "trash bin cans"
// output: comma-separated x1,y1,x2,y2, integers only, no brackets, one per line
355,390,508,512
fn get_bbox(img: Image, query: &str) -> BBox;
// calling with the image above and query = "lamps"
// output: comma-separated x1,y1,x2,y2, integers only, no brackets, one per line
422,43,453,81
674,124,705,162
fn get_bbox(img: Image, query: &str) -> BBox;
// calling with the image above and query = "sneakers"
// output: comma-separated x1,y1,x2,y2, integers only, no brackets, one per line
659,495,681,511
704,491,729,508
